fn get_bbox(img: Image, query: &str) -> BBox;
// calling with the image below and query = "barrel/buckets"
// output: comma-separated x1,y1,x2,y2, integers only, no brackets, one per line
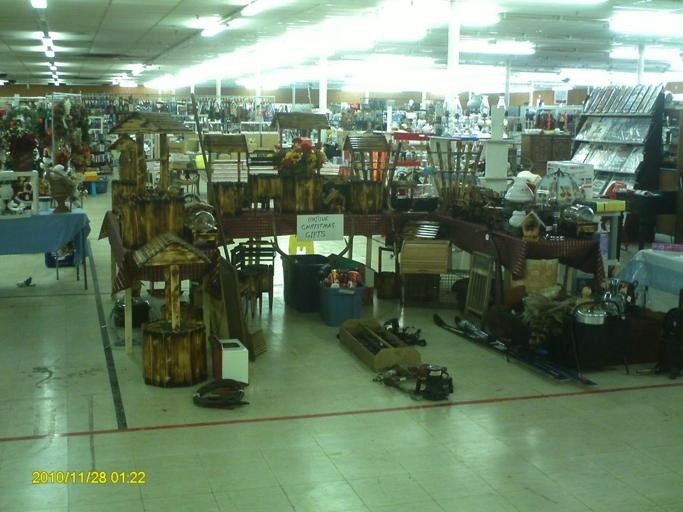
375,243,400,299
562,203,593,223
569,317,608,370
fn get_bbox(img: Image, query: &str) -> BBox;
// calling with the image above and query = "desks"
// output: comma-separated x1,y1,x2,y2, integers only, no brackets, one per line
210,209,606,307
617,248,683,311
0,209,91,290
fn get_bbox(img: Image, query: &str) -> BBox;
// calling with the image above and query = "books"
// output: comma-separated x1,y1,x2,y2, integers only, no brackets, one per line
209,156,342,184
569,83,665,198
652,243,683,251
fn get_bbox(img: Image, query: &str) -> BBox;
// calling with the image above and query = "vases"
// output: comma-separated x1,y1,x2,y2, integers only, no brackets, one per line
13,150,33,172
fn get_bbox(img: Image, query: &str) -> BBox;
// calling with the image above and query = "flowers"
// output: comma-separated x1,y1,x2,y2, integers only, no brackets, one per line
0,127,36,152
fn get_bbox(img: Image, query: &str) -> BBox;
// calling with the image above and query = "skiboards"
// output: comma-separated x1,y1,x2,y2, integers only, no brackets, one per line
435,313,599,388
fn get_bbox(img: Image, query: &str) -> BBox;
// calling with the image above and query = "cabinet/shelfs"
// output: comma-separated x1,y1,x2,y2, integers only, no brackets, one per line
521,93,683,241
